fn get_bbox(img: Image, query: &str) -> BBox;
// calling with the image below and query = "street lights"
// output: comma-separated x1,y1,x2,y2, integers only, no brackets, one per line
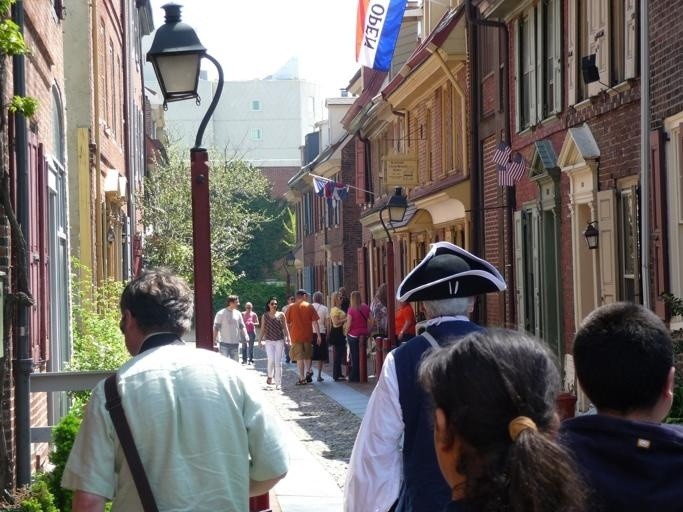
281,251,295,295
147,3,223,350
379,187,408,348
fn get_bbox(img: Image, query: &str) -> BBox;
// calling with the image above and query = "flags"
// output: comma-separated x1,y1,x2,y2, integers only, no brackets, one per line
306,173,379,203
355,0,408,72
491,142,533,187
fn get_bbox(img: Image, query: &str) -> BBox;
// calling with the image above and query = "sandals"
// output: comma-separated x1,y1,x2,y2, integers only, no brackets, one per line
306,376,313,382
294,379,306,385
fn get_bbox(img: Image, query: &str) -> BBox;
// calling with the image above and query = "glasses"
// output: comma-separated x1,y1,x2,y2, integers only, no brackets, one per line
268,302,278,306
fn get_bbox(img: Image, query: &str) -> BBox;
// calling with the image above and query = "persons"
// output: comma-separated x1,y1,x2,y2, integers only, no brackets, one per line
395,301,416,340
417,325,582,511
286,290,322,385
343,242,508,512
339,287,349,312
212,295,250,363
240,302,260,365
551,301,682,512
60,272,289,511
282,295,295,311
258,297,292,390
369,283,388,337
330,292,348,382
343,291,372,382
307,292,330,381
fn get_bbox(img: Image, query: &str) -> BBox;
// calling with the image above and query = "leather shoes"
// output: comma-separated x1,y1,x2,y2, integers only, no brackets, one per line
317,377,324,382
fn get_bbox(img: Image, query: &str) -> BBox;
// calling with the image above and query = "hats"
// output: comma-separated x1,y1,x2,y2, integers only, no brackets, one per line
394,241,507,303
296,289,307,295
